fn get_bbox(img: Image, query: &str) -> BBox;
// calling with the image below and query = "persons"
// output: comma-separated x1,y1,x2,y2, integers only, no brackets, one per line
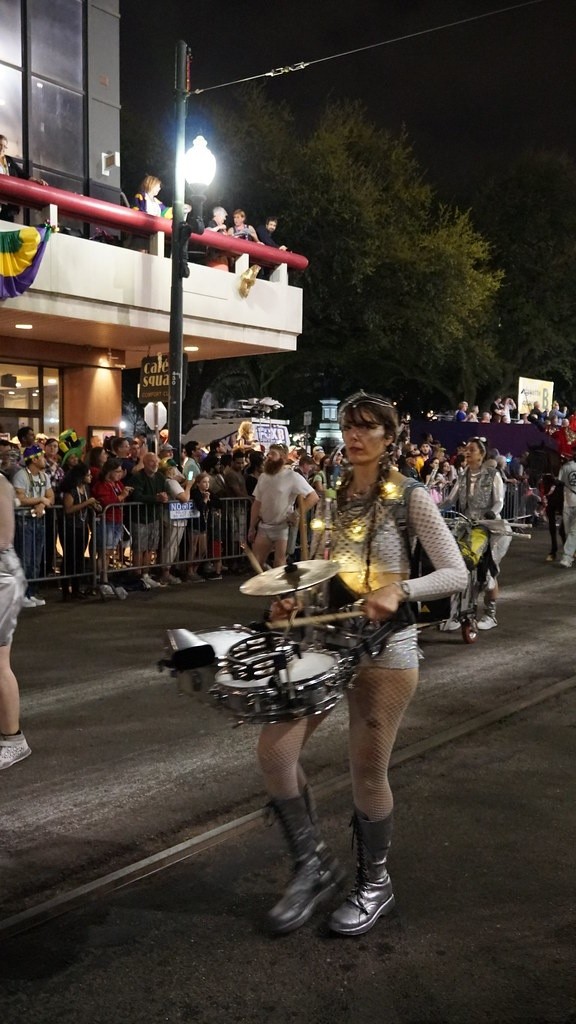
0,473,33,771
0,425,549,608
0,134,50,220
133,177,288,280
256,392,470,935
446,437,511,635
552,446,576,568
456,394,571,428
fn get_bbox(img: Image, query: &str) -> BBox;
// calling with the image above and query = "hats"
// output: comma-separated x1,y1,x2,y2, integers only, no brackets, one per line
159,457,178,468
57,441,83,469
60,429,85,449
159,444,178,451
24,445,46,461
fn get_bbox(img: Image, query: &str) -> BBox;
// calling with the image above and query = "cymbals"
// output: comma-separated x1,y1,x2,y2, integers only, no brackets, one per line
225,631,301,681
239,558,341,598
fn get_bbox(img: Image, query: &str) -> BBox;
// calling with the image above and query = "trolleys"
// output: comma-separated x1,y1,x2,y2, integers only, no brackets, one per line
408,507,488,643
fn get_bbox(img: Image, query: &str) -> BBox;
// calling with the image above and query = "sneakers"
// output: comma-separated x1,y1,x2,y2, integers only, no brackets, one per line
0,729,31,770
23,596,36,607
29,596,45,606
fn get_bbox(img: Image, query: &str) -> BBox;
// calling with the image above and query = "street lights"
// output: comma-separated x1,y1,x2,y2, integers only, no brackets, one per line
168,40,220,471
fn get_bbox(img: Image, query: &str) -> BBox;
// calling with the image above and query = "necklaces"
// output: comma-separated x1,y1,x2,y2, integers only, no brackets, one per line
77,485,89,522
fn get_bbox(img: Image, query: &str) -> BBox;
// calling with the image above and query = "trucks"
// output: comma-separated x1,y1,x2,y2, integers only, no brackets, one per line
178,396,291,457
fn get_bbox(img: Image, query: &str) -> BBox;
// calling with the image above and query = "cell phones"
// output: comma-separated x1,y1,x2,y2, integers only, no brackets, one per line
187,471,194,480
31,508,46,517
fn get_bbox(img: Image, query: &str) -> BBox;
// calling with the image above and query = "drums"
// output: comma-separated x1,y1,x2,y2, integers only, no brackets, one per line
174,624,268,702
212,647,344,725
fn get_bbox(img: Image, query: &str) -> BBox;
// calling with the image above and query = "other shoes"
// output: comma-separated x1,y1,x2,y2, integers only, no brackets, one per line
163,574,182,584
187,573,205,583
101,585,115,594
144,577,159,588
208,573,223,580
560,559,572,568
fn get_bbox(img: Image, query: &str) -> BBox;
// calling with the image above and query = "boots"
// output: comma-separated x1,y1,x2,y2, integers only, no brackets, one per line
477,597,497,630
328,809,395,936
260,782,347,936
